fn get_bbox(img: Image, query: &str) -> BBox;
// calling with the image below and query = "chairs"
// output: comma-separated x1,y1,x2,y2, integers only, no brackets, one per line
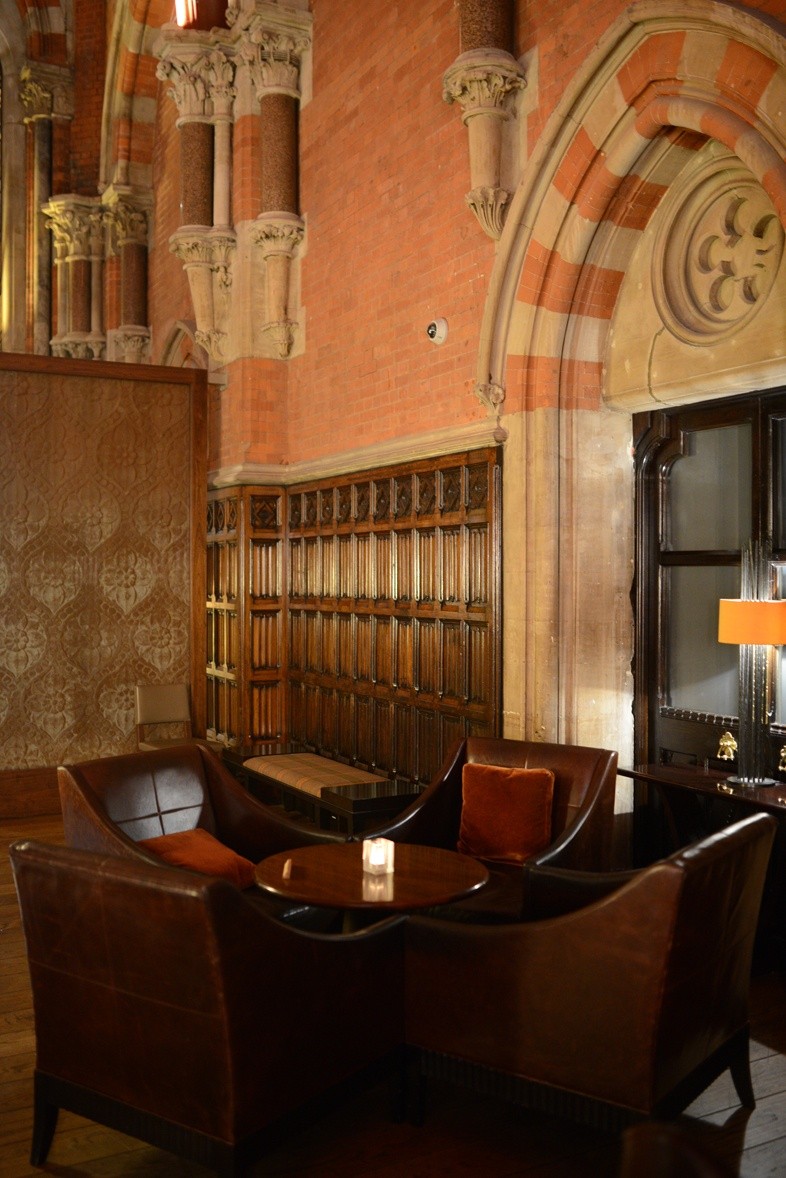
134,684,226,752
7,735,776,1178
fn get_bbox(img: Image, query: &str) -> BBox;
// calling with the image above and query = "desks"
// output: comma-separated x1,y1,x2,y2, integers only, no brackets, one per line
254,841,489,934
616,764,786,850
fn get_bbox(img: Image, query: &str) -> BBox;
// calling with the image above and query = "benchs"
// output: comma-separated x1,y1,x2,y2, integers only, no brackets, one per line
222,743,420,835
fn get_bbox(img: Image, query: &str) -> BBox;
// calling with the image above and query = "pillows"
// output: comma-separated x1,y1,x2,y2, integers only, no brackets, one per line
457,763,555,863
139,828,257,891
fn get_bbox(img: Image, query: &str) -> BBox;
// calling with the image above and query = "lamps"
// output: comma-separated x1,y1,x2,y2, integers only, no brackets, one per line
717,599,786,646
